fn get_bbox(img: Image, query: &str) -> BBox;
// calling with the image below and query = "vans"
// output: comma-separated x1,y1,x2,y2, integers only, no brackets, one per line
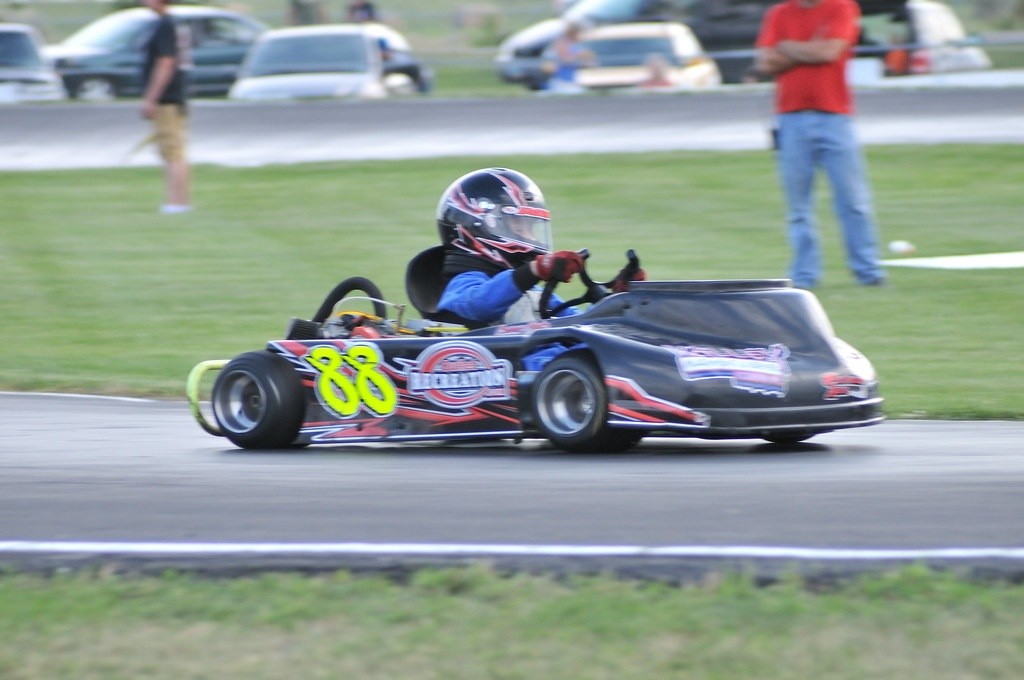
494,0,783,90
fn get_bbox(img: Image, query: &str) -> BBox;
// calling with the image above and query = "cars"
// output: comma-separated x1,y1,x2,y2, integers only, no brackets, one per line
846,0,993,73
228,21,430,101
547,21,724,92
0,24,69,102
52,5,267,101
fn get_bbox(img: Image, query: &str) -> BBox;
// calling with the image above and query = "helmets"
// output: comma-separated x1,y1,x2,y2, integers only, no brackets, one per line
434,168,551,270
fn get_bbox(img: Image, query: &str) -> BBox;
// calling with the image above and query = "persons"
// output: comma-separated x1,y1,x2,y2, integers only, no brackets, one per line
541,17,594,92
291,0,322,24
635,53,679,88
754,0,887,289
436,168,646,330
348,0,374,23
139,0,195,214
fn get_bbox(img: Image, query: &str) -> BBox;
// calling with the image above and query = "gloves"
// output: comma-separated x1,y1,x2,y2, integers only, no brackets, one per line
536,250,587,284
612,269,646,292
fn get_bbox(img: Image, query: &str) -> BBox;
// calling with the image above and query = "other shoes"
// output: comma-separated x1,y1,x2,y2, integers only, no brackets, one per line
161,204,189,213
